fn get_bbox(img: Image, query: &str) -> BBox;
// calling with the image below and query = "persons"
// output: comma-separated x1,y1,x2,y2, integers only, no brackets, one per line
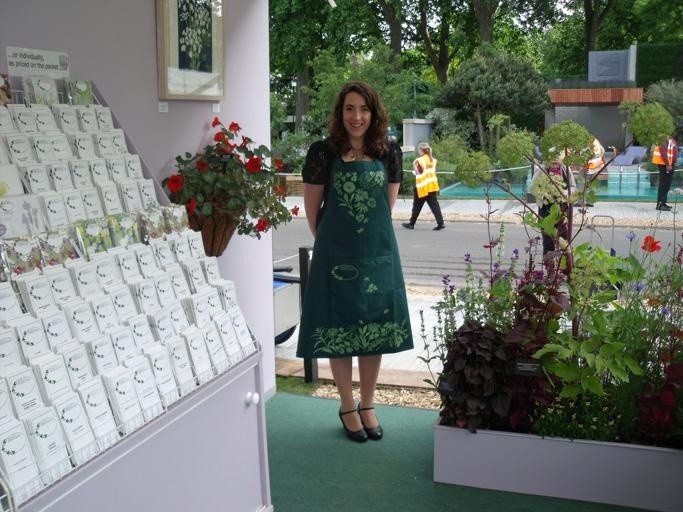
401,141,446,230
585,135,603,182
534,159,576,253
652,136,678,211
296,79,414,442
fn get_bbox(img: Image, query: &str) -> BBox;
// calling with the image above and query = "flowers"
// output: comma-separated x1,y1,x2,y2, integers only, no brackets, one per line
159,117,299,240
418,186,682,451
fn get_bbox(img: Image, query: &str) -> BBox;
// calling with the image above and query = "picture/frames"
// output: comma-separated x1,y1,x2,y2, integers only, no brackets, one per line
154,1,227,101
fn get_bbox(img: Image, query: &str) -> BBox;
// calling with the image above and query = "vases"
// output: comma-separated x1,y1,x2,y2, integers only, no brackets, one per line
196,198,243,258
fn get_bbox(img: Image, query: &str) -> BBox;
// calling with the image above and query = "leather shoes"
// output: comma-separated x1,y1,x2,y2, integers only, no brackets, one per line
656,201,672,211
434,224,445,230
358,402,383,439
339,406,368,442
402,223,414,229
